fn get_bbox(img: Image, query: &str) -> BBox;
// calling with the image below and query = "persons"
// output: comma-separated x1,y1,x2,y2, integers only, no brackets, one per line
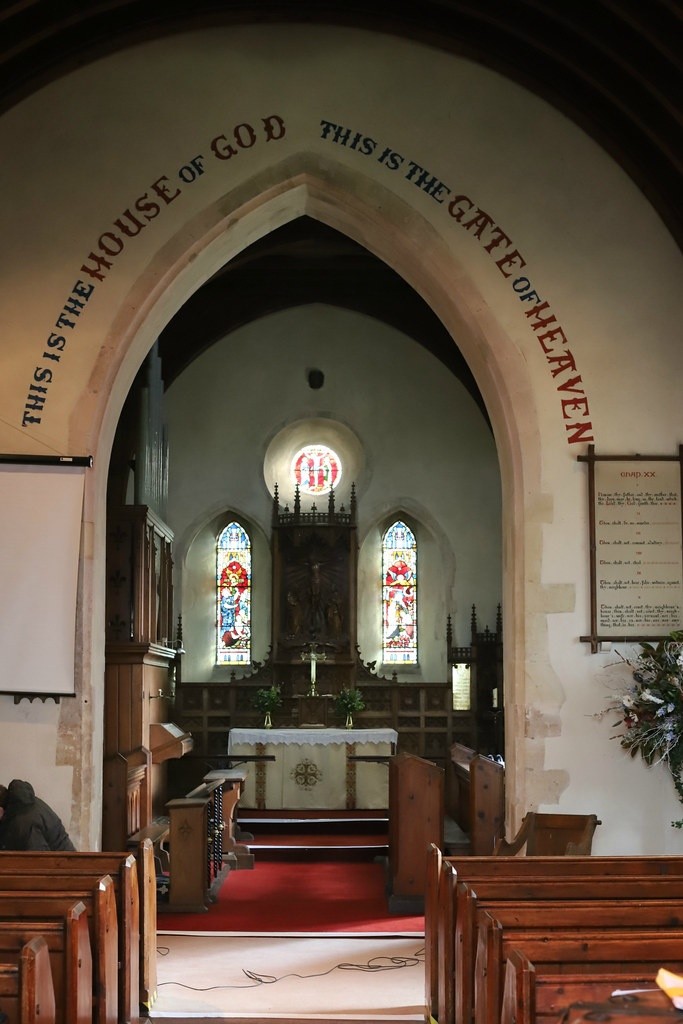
0,779,78,852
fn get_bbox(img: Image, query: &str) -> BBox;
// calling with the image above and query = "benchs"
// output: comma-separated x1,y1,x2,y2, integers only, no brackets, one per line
0,836,156,1024
443,811,470,855
127,816,171,874
423,840,683,1024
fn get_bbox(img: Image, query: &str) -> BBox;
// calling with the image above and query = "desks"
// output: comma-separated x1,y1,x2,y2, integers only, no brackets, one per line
226,728,398,810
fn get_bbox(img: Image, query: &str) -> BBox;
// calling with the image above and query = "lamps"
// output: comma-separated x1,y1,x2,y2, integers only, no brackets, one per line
163,638,187,655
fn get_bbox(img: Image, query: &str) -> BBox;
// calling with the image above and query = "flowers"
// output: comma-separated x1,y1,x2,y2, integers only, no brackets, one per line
586,630,683,835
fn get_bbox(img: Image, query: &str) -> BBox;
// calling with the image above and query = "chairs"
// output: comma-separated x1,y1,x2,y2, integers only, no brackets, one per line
492,812,602,856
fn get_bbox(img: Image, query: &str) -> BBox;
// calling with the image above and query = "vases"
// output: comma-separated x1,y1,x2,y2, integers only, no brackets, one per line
346,714,354,729
263,711,273,729
